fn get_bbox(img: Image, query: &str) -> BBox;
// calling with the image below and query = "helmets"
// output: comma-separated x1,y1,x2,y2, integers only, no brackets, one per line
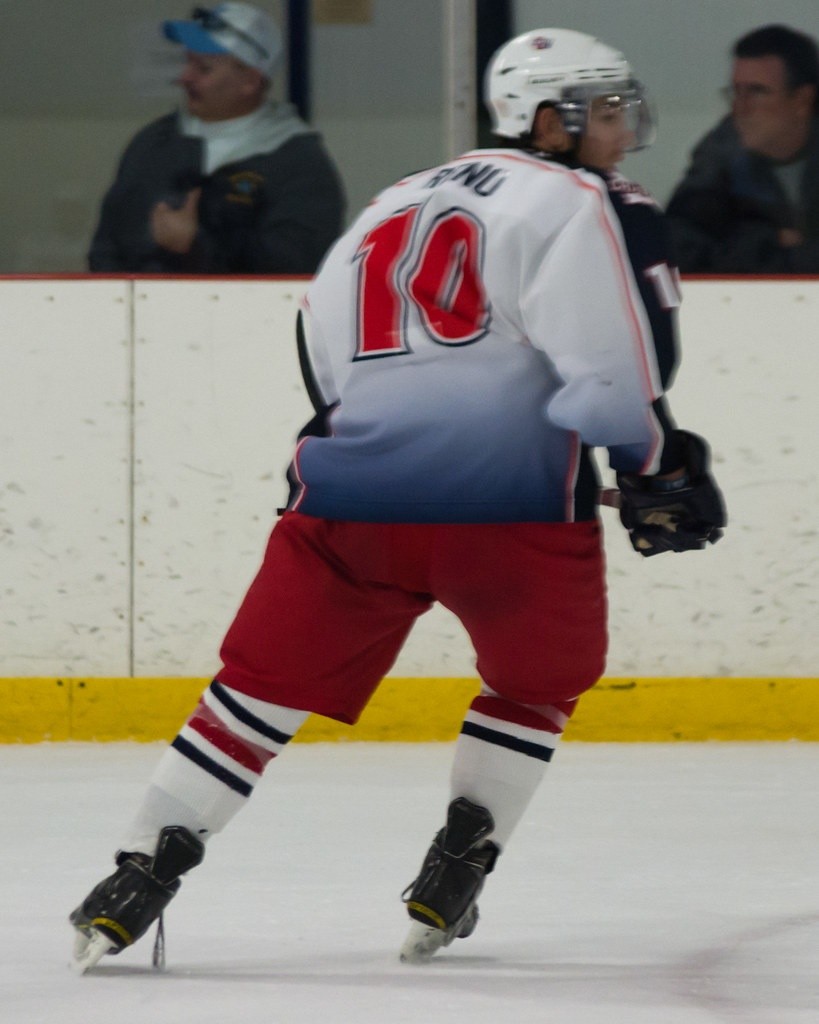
483,28,655,151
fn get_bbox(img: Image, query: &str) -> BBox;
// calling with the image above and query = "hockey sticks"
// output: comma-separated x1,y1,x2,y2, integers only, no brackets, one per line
597,484,622,509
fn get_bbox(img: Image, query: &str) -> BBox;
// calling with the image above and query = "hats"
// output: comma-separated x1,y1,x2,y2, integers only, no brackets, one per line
161,3,281,84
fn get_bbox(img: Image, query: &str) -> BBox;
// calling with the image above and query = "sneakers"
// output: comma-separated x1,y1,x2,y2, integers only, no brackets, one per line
399,798,500,930
69,823,206,971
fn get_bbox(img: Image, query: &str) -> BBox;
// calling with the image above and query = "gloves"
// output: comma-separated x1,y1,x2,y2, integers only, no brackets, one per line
616,430,727,557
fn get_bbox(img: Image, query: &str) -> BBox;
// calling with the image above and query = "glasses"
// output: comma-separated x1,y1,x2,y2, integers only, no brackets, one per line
191,7,270,60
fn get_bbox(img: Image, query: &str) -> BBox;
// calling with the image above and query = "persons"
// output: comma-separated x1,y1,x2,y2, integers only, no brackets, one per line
663,25,818,278
86,1,347,280
68,23,730,969
